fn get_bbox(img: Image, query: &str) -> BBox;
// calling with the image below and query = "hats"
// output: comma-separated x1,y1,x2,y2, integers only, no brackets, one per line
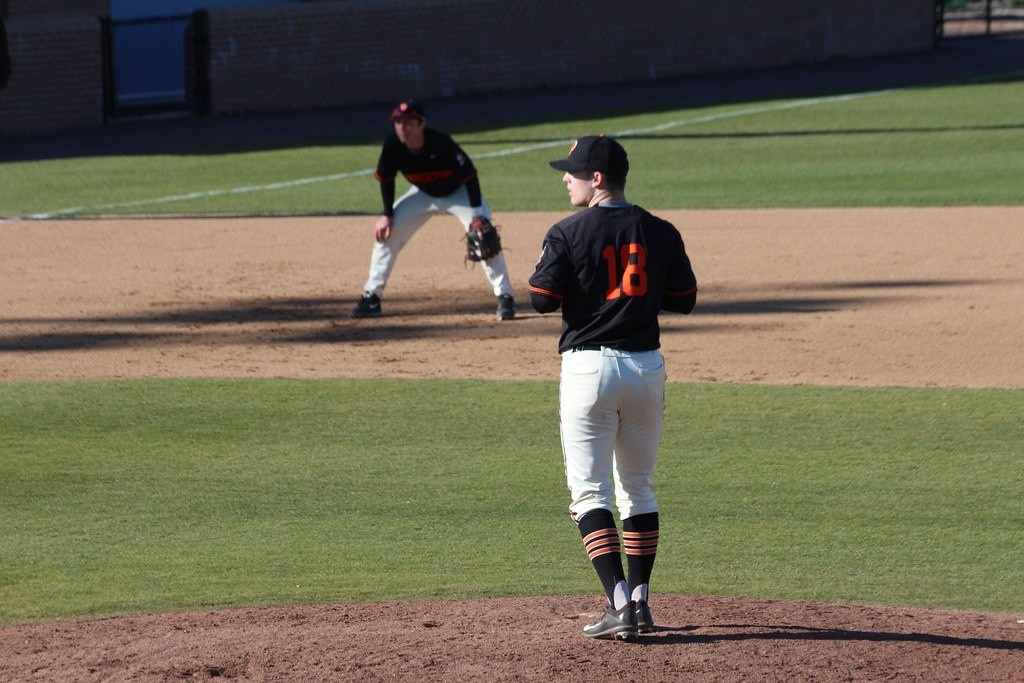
389,99,425,120
550,134,629,177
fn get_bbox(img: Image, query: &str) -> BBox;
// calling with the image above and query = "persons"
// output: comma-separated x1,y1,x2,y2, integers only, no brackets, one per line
529,135,699,641
349,98,522,323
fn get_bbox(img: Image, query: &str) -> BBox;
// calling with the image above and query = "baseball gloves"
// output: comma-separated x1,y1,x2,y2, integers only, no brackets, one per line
459,215,512,270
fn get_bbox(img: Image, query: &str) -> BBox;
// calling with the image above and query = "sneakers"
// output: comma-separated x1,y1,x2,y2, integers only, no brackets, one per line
497,293,515,320
583,601,654,640
351,294,383,318
